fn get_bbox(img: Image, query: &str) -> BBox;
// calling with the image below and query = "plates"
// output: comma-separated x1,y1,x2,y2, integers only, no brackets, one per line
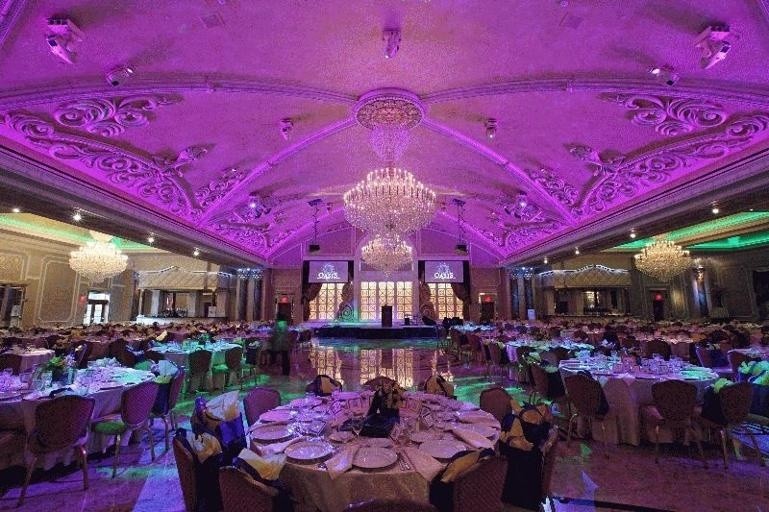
250,391,499,470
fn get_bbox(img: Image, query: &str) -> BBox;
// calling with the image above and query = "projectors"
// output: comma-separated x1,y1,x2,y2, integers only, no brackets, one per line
456,244,466,249
309,245,319,250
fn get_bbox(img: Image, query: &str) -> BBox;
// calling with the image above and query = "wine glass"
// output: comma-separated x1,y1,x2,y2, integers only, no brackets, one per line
578,351,684,376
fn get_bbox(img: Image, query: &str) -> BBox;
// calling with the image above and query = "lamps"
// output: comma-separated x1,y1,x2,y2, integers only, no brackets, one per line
344,120,437,233
361,233,412,276
633,234,693,283
69,231,128,283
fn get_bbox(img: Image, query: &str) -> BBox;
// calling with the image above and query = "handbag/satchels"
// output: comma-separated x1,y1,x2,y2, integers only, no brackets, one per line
359,390,397,438
